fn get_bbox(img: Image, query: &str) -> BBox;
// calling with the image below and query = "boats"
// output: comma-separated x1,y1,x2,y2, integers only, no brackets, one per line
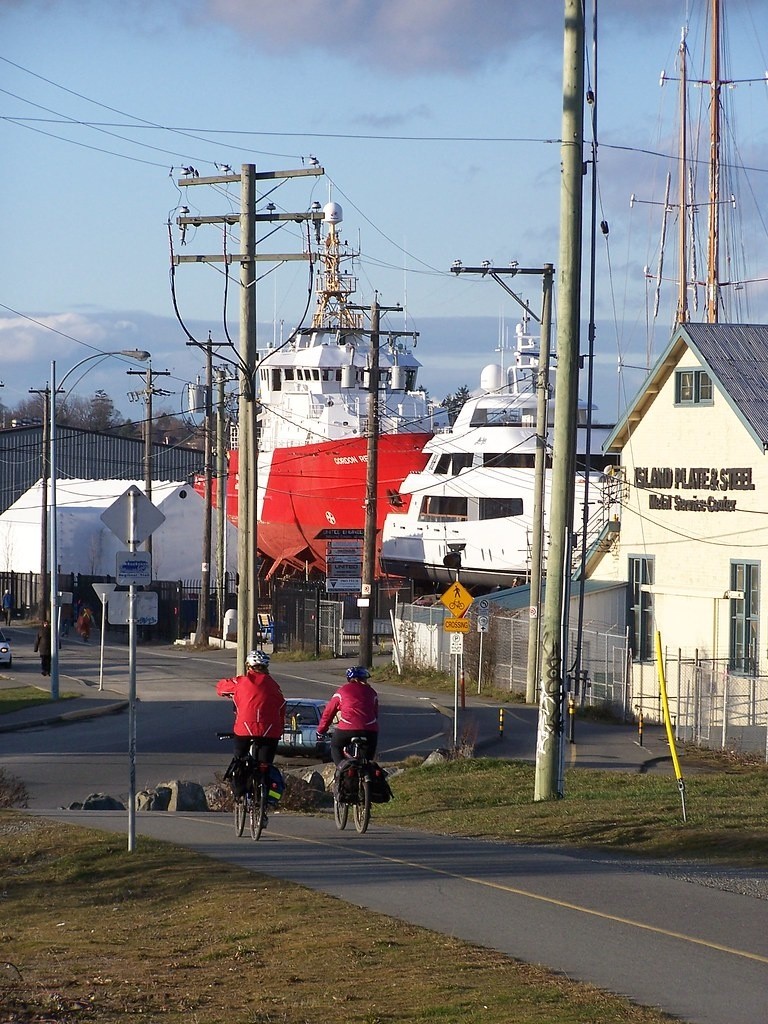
194,200,442,577
380,365,618,588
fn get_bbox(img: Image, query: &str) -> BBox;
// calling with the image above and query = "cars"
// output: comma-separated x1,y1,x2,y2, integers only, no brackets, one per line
0,630,12,668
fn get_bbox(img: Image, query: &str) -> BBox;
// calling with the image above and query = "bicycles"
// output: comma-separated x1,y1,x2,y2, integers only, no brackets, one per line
216,731,265,841
322,733,371,834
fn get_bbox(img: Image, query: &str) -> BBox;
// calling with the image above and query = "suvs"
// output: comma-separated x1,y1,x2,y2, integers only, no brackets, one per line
278,699,342,764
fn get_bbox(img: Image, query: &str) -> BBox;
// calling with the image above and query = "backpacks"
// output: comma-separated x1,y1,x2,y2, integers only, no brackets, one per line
252,763,285,806
332,757,359,805
365,761,395,803
222,755,252,805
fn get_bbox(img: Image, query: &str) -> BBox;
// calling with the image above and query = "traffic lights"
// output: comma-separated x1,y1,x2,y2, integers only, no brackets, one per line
442,553,461,569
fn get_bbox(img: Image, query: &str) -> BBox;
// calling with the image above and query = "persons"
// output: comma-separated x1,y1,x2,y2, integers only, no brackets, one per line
78,602,91,643
2,590,14,626
215,650,286,767
60,604,74,638
318,665,380,767
34,621,62,676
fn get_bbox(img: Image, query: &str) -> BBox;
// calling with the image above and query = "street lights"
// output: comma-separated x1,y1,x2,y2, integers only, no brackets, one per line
51,349,151,699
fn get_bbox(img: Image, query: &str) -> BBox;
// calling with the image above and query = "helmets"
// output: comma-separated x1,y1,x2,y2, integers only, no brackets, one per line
245,649,270,667
346,665,372,682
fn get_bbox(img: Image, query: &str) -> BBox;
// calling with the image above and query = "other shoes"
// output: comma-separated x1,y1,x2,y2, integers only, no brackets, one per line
62,633,68,638
41,671,50,676
